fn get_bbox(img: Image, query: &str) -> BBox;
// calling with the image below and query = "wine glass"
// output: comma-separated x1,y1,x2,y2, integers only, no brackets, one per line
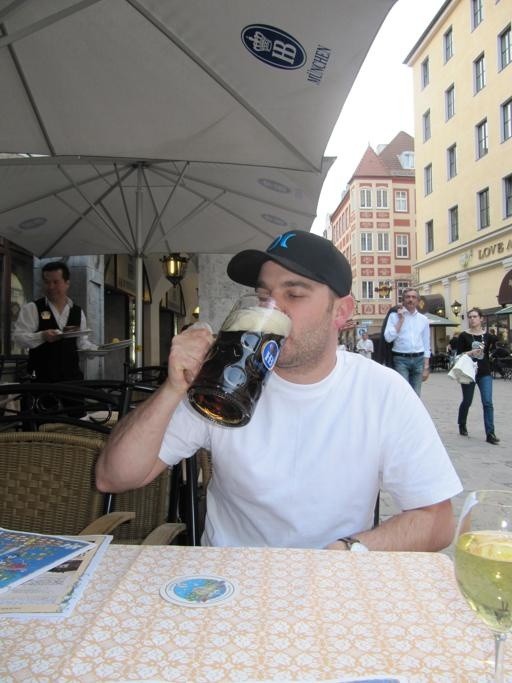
454,490,512,683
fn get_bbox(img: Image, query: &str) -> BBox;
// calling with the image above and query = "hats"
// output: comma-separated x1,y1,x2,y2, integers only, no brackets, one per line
227,229,352,297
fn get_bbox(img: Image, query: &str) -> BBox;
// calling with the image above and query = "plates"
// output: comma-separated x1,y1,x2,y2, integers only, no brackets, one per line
58,330,93,338
77,337,136,355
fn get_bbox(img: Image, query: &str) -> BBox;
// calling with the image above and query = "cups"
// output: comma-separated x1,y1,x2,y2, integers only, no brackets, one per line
182,293,293,429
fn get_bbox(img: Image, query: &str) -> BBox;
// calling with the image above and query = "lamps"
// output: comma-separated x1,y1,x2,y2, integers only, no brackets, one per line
158,252,189,288
451,300,465,320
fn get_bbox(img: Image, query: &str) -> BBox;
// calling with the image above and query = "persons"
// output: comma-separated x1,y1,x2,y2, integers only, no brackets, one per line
95,229,466,552
383,288,431,397
356,333,375,359
448,308,507,443
10,263,100,431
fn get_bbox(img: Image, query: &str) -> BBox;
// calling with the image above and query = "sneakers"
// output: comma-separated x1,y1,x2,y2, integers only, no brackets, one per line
460,426,468,436
487,434,500,443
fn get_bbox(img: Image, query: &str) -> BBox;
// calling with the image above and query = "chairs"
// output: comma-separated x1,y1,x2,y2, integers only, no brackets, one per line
489,357,512,380
428,353,451,373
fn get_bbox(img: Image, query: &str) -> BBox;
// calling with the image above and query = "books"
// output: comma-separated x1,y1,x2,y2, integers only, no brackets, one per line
0,526,114,621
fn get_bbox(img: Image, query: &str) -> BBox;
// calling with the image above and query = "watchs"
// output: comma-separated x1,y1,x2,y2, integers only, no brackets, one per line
337,537,368,552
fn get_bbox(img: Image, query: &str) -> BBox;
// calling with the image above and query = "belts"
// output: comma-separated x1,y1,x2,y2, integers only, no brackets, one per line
392,351,425,358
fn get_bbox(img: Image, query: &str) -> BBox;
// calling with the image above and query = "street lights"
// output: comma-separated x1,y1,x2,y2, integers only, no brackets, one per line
450,298,462,331
